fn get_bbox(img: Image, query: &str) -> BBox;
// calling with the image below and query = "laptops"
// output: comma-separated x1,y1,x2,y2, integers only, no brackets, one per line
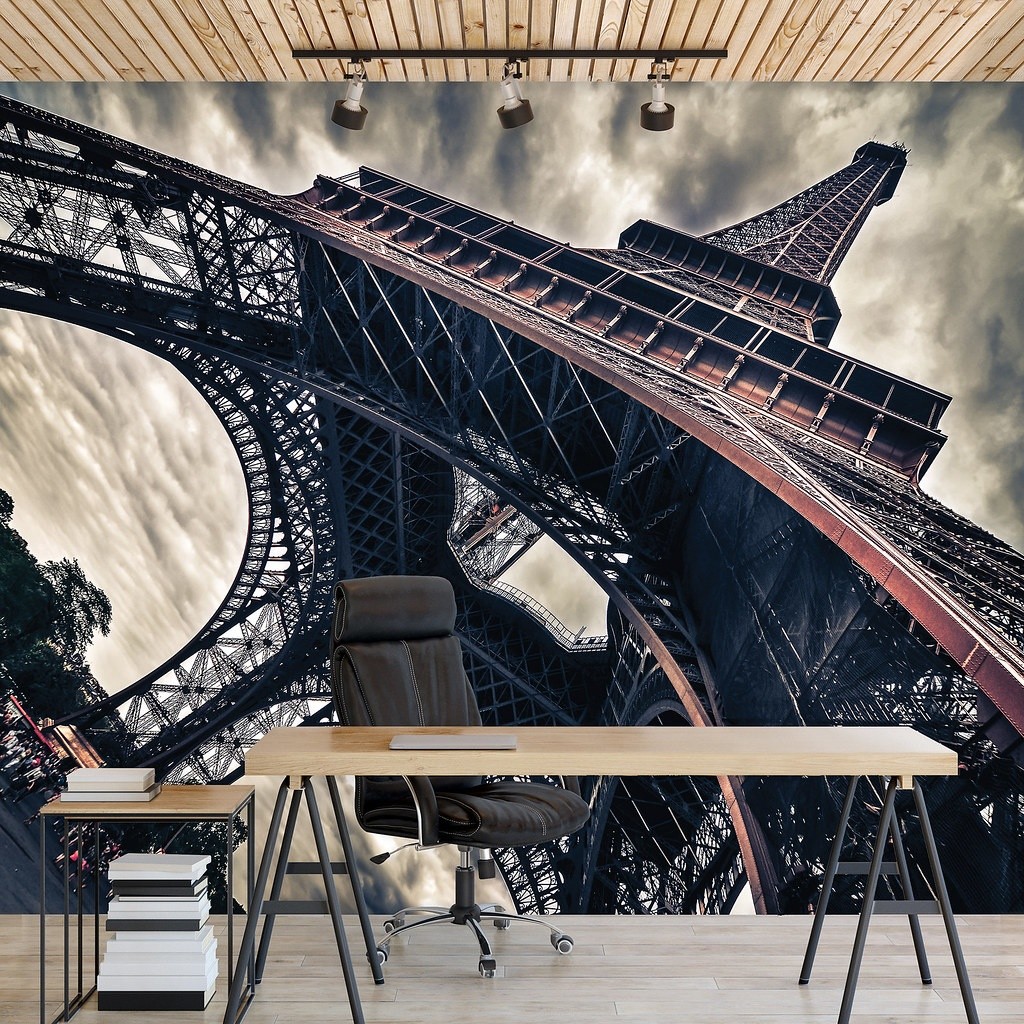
389,734,517,751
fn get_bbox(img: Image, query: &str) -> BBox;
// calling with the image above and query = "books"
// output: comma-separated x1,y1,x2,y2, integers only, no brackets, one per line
97,853,219,1012
61,768,162,802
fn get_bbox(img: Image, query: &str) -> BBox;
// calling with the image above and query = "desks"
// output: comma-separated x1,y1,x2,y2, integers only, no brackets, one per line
224,726,979,1024
39,785,255,1024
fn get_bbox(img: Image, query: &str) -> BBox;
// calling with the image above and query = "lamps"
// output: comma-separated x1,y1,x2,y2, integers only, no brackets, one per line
332,57,368,131
496,55,534,129
641,57,674,132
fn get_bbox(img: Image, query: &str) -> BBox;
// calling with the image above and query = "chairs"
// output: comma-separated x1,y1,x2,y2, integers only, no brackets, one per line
329,577,589,978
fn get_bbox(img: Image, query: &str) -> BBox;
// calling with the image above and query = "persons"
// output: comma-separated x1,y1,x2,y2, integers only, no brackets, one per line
0,698,122,898
